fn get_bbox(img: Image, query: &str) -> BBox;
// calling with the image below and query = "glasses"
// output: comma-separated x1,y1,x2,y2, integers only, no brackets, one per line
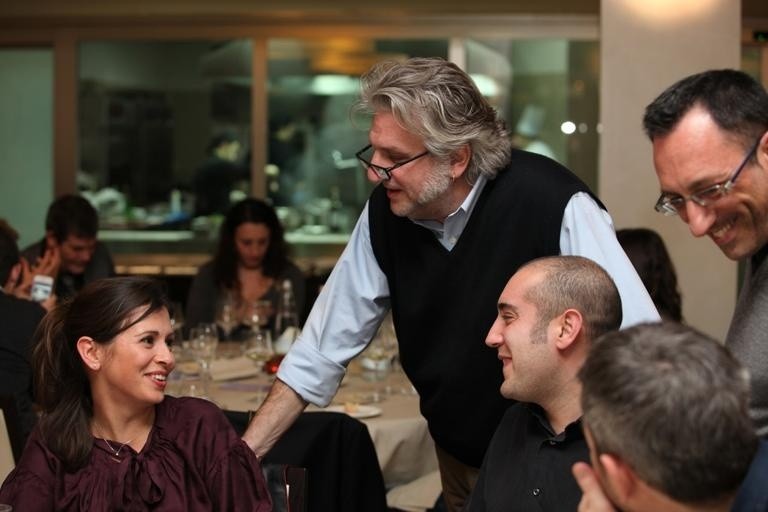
355,145,429,181
655,138,760,217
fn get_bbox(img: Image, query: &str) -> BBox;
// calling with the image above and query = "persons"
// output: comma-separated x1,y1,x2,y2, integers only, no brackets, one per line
0,219,65,462
570,319,755,512
642,69,767,512
20,192,113,303
462,254,622,512
184,197,307,340
233,53,663,512
0,278,276,512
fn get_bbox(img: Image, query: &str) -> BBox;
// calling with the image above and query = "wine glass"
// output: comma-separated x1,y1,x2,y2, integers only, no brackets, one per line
167,296,403,397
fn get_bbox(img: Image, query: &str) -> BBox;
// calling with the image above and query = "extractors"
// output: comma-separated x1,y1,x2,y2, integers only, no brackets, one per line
195,39,515,103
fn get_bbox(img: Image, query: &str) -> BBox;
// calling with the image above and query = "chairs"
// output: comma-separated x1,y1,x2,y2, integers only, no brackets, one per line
216,408,389,512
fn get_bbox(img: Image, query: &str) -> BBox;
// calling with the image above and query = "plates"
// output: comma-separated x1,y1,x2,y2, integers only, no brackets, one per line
318,406,382,420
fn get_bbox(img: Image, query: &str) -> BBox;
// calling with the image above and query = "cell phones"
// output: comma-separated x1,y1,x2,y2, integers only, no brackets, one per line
30,274,55,304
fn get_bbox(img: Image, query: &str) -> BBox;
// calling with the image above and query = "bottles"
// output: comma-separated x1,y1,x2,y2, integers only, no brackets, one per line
272,277,300,353
